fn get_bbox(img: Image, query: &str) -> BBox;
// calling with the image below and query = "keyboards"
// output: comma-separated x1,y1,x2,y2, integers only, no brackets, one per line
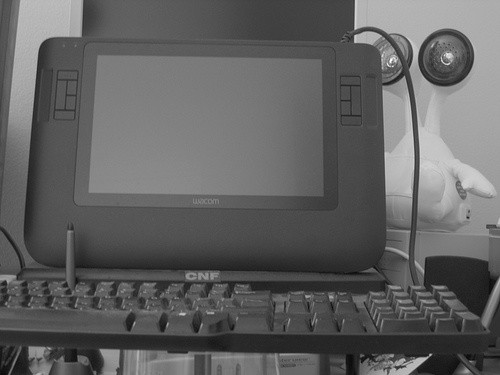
2,272,490,356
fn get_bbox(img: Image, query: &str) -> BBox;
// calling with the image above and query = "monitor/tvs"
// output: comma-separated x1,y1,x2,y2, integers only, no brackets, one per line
23,38,387,270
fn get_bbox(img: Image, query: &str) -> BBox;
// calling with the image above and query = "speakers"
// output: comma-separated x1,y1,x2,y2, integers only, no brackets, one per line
422,257,489,375
